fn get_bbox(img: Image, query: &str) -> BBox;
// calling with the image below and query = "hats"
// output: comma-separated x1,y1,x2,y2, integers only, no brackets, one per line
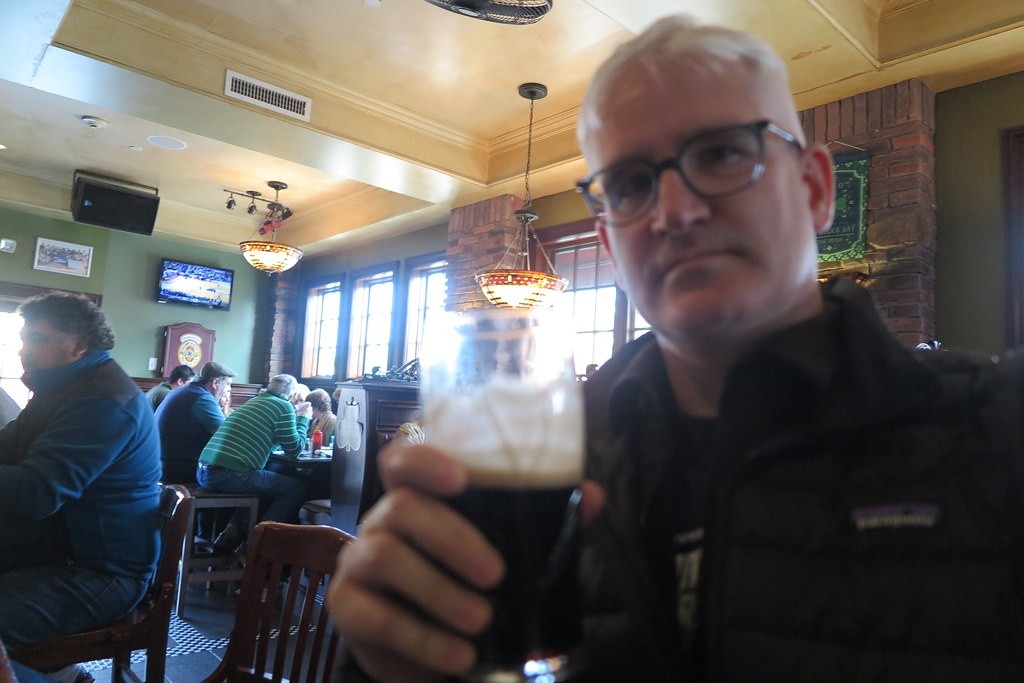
201,362,235,378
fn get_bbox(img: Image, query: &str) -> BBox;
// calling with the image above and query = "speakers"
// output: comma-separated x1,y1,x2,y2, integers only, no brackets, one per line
72,177,160,235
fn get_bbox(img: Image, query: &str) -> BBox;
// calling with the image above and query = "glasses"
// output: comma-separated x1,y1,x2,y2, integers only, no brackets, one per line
575,118,804,228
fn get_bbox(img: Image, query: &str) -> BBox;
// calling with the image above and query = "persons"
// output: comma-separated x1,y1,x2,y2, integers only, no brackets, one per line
306,388,337,447
39,242,88,266
392,418,423,442
0,291,163,683
220,391,235,417
155,362,239,553
146,364,196,412
291,384,310,410
197,374,313,569
327,18,1024,683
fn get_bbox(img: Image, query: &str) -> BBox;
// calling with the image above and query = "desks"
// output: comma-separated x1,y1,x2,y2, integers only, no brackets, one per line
269,446,332,500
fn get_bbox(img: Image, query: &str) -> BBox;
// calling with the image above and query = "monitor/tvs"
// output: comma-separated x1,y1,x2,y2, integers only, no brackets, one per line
157,258,234,311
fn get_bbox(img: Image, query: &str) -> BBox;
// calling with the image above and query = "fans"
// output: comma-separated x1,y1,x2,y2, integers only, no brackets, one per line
426,0,554,25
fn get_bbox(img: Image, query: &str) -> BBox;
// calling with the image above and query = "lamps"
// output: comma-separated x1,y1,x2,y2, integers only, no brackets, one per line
222,188,292,220
238,181,304,277
472,81,570,314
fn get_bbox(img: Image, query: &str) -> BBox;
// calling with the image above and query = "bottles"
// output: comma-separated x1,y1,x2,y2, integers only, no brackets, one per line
312,429,323,454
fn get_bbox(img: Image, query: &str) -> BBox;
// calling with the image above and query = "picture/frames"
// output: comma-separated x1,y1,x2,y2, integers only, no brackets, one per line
0,238,18,253
33,237,94,279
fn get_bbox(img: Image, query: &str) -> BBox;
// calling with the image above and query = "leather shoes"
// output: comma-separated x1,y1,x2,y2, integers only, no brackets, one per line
208,532,247,556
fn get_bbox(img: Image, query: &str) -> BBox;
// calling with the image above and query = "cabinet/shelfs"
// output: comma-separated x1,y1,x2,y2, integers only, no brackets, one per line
327,382,424,536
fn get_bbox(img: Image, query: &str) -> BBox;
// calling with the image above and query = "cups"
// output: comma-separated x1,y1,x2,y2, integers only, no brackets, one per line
416,308,587,683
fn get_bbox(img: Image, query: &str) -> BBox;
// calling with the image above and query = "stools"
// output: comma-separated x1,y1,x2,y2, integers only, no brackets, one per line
297,499,329,593
174,489,258,617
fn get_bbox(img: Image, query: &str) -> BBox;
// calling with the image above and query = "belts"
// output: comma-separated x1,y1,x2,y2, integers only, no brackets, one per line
198,462,225,471
160,455,196,462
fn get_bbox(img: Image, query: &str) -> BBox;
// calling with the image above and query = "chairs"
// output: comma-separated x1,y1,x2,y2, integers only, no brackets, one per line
197,520,357,683
6,483,195,682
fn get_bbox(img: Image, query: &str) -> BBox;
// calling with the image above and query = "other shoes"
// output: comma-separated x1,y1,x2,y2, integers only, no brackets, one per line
45,664,95,683
